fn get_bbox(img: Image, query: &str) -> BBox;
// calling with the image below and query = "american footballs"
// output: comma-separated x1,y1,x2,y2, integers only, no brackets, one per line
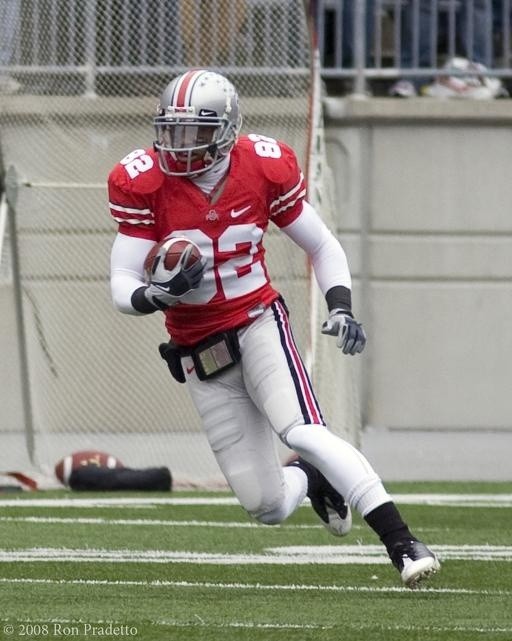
55,450,122,488
144,235,201,278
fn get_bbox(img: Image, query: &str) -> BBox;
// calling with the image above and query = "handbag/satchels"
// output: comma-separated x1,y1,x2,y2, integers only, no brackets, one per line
430,58,501,99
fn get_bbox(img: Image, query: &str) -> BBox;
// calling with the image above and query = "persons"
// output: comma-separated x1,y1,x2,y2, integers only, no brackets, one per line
0,0,509,98
108,70,442,587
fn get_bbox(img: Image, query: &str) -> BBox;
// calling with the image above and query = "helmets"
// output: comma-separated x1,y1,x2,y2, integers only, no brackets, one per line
153,69,243,177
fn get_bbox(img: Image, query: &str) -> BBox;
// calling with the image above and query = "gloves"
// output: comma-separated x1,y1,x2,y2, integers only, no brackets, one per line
321,307,366,355
144,244,208,309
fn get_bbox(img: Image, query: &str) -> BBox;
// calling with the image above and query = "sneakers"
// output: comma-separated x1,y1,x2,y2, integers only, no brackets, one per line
388,79,416,98
0,73,24,95
286,453,352,536
390,540,441,589
